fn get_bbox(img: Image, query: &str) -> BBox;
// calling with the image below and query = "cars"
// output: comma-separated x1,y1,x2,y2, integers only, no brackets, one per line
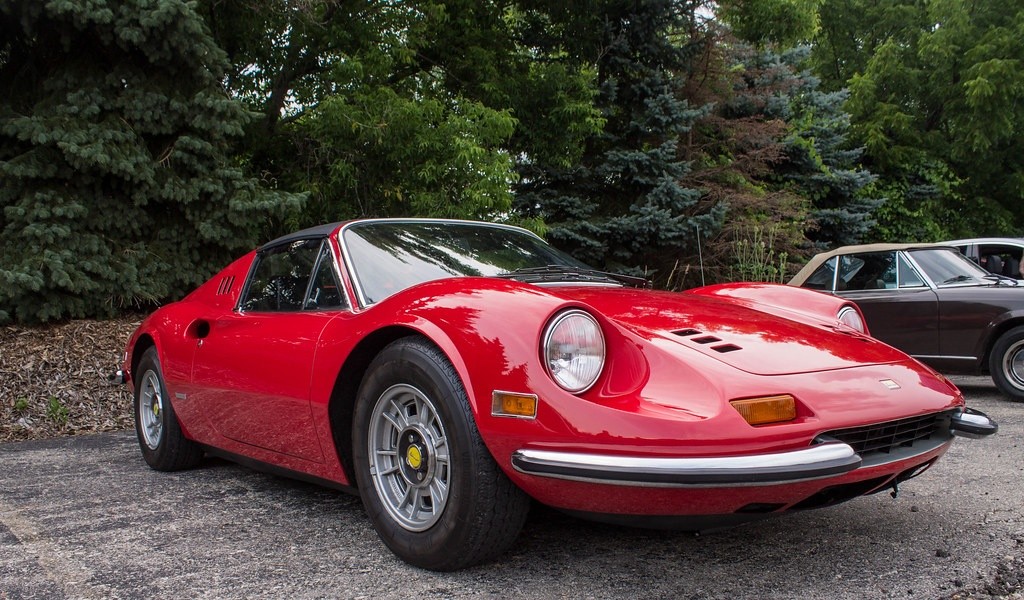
786,245,1024,402
923,237,1024,280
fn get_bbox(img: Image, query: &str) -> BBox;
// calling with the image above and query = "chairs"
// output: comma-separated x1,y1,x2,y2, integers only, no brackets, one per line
267,275,328,309
985,255,1002,275
824,278,848,290
864,279,886,289
1004,260,1020,277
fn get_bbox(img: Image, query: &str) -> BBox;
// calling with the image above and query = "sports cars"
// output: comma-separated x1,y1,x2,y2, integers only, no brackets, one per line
107,218,999,573
